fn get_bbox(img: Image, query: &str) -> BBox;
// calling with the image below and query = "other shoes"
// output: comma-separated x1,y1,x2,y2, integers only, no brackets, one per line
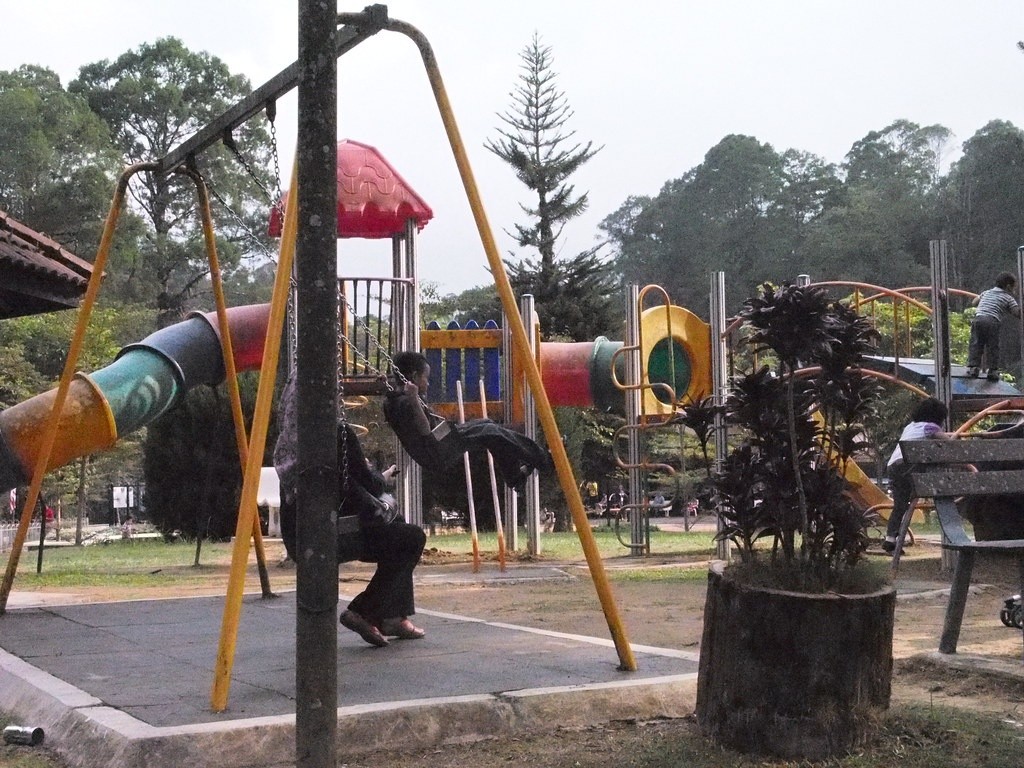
970,365,980,377
881,541,906,555
372,614,425,639
540,435,567,471
508,463,537,493
338,608,391,647
987,369,1002,381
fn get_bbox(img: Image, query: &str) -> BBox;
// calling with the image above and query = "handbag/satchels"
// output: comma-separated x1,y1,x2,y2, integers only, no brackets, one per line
353,479,399,528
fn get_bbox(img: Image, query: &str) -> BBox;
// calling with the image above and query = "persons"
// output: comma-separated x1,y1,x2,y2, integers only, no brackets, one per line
121,516,133,539
273,365,427,648
881,399,980,554
967,271,1020,381
382,351,568,493
41,504,55,538
539,479,700,534
